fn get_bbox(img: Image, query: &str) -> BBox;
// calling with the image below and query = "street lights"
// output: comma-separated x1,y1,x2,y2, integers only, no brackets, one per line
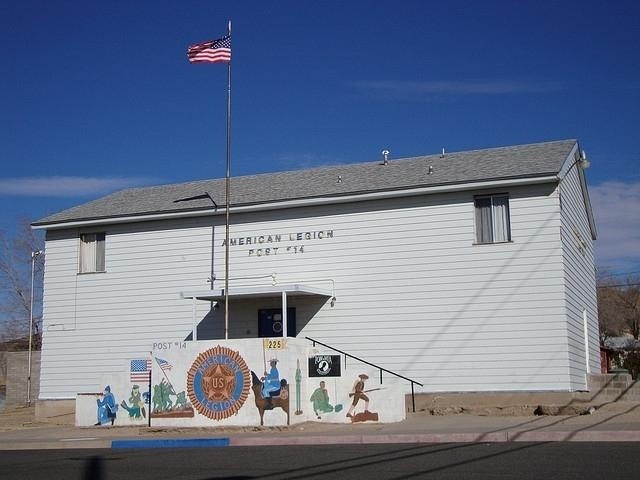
26,250,45,407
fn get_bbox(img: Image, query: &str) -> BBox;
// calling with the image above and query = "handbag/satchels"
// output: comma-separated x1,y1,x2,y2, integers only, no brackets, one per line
110,404,119,414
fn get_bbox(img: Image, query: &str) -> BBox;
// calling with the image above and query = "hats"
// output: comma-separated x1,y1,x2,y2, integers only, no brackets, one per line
132,384,139,389
104,385,111,391
358,374,369,379
267,356,280,362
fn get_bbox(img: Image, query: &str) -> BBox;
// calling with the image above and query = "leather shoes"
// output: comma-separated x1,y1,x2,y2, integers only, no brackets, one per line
364,410,372,414
345,412,354,418
111,418,116,425
94,422,102,426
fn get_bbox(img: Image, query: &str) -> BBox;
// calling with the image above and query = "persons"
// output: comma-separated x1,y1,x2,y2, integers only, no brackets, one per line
260,359,281,408
94,385,116,426
152,377,186,414
129,385,143,418
295,358,302,415
346,374,371,418
310,381,333,419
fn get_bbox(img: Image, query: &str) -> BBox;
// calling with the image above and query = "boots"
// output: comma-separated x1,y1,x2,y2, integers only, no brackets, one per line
262,397,273,409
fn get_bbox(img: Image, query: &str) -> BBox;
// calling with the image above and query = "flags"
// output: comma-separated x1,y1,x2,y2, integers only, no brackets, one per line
156,358,173,371
186,35,232,64
130,360,152,382
